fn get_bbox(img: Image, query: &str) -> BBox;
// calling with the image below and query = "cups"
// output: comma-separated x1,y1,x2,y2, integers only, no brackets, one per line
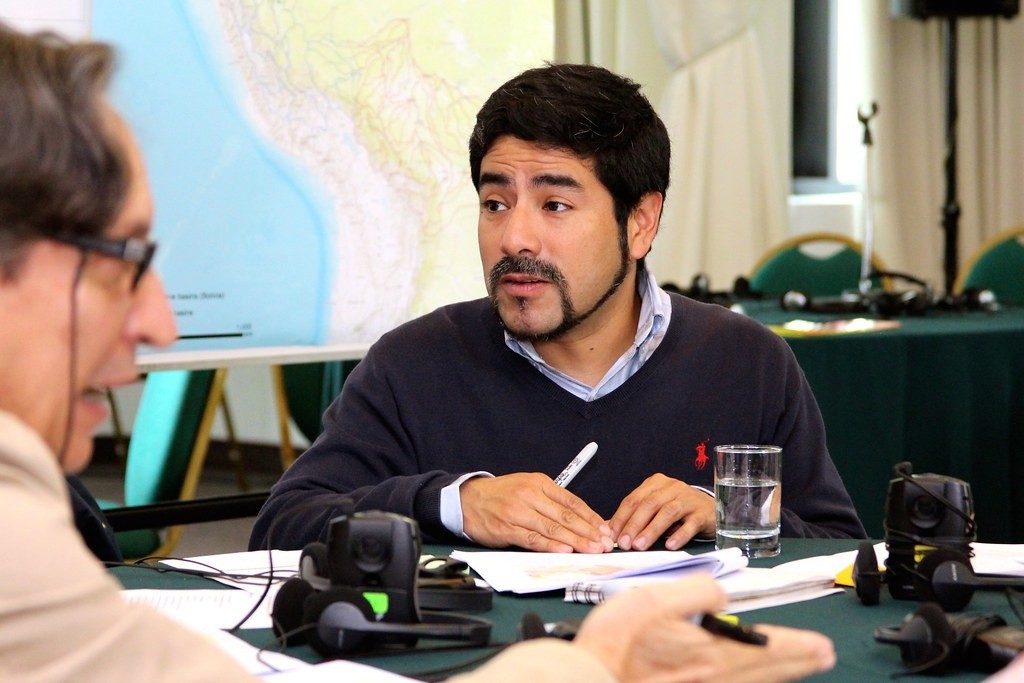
712,443,783,560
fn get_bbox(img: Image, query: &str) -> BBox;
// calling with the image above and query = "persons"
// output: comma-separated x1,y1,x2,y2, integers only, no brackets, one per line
249,63,867,549
0,24,839,683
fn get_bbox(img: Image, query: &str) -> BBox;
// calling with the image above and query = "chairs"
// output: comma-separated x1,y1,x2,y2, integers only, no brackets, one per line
748,231,891,301
99,360,359,564
952,215,1024,304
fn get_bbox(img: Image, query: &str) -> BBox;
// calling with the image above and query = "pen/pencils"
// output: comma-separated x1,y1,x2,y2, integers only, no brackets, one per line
693,611,768,644
553,442,598,489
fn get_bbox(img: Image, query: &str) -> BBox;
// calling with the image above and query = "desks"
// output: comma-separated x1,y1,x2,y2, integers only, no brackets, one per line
95,535,1024,683
741,301,1024,547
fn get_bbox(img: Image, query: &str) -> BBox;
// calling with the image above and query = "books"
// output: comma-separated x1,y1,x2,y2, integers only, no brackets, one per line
564,562,847,615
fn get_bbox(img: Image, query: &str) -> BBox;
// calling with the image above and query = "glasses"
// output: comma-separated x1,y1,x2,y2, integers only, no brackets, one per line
31,221,156,290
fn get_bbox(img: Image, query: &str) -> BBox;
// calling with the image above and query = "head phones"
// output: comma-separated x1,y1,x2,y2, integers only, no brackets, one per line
270,541,495,662
852,540,1024,678
